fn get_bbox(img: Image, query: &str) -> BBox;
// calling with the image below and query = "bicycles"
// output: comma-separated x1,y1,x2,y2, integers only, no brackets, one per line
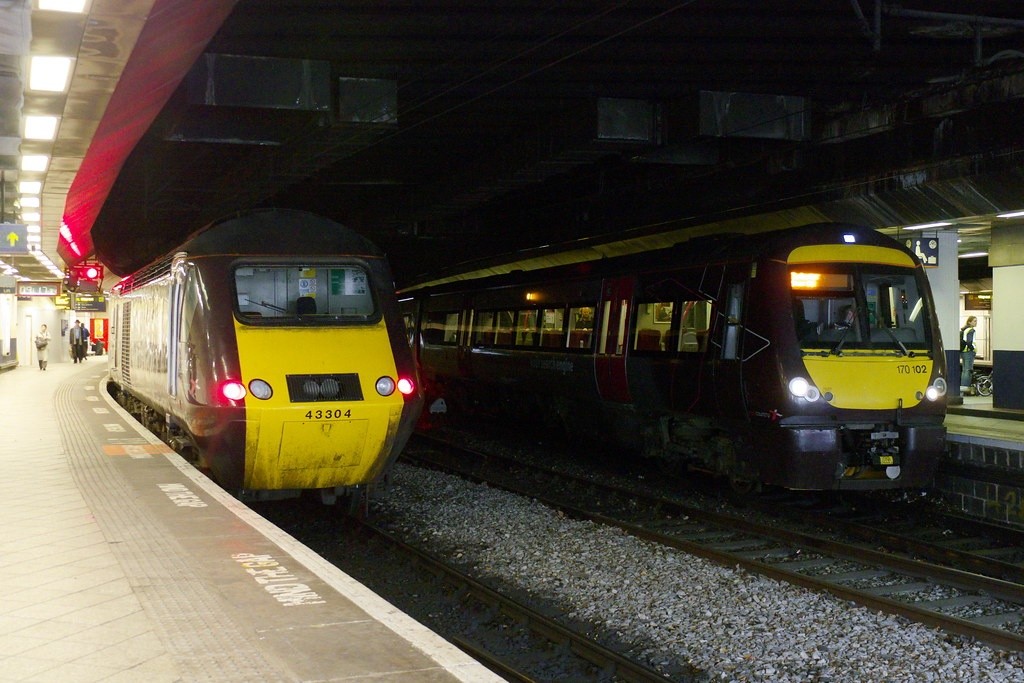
972,369,994,396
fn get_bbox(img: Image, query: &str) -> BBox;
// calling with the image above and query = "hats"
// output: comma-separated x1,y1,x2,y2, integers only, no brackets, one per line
74,320,80,323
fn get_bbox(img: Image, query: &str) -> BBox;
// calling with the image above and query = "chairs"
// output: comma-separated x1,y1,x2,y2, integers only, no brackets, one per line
454,329,710,352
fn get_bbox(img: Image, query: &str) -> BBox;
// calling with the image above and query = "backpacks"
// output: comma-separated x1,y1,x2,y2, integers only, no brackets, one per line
959,327,972,352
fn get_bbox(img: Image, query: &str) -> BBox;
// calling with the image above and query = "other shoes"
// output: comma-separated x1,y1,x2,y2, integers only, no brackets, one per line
85,357,87,360
963,393,975,397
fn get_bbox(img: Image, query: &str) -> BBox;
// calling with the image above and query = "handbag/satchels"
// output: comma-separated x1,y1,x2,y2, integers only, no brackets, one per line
36,340,48,348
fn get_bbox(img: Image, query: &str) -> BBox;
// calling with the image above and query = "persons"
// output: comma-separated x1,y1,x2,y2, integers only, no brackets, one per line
69,320,90,363
575,307,593,329
35,324,51,370
959,316,978,396
95,338,105,355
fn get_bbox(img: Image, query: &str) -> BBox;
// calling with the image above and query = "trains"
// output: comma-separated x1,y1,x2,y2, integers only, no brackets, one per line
108,206,426,520
397,221,949,520
